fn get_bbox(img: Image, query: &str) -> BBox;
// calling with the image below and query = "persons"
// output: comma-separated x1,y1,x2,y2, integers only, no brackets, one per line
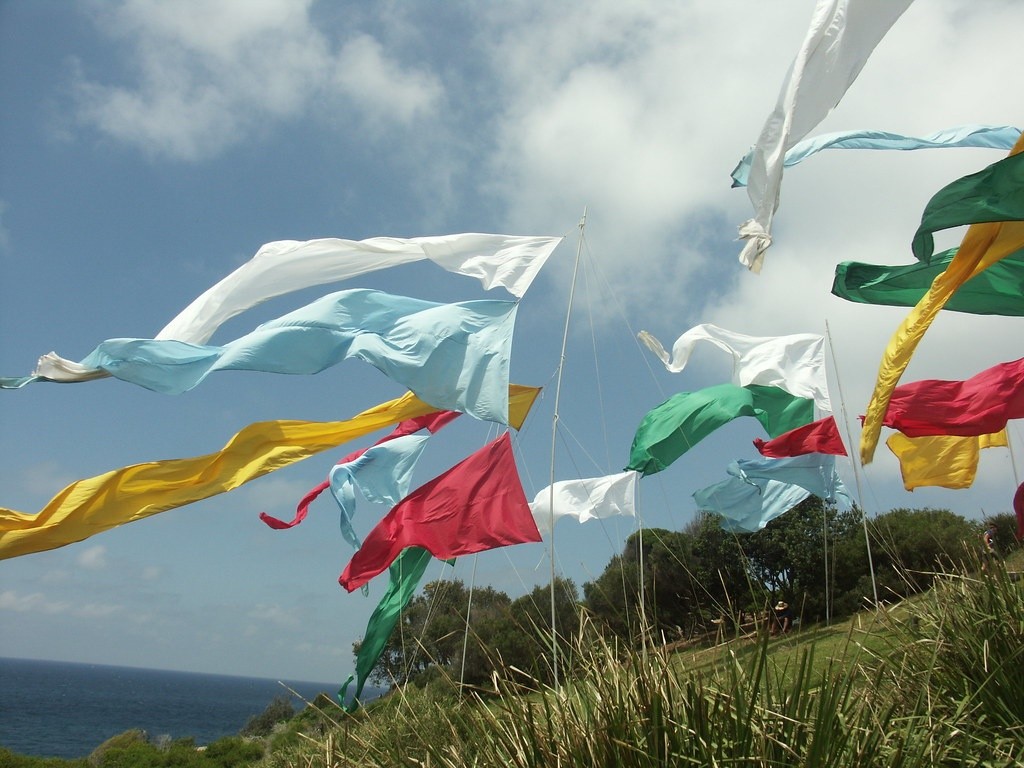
981,524,1002,572
769,601,790,635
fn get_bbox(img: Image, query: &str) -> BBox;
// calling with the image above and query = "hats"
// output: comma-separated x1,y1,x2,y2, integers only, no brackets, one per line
775,601,788,610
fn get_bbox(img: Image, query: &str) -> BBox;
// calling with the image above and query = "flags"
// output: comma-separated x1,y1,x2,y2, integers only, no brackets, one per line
0,234,641,719
623,0,1024,540
